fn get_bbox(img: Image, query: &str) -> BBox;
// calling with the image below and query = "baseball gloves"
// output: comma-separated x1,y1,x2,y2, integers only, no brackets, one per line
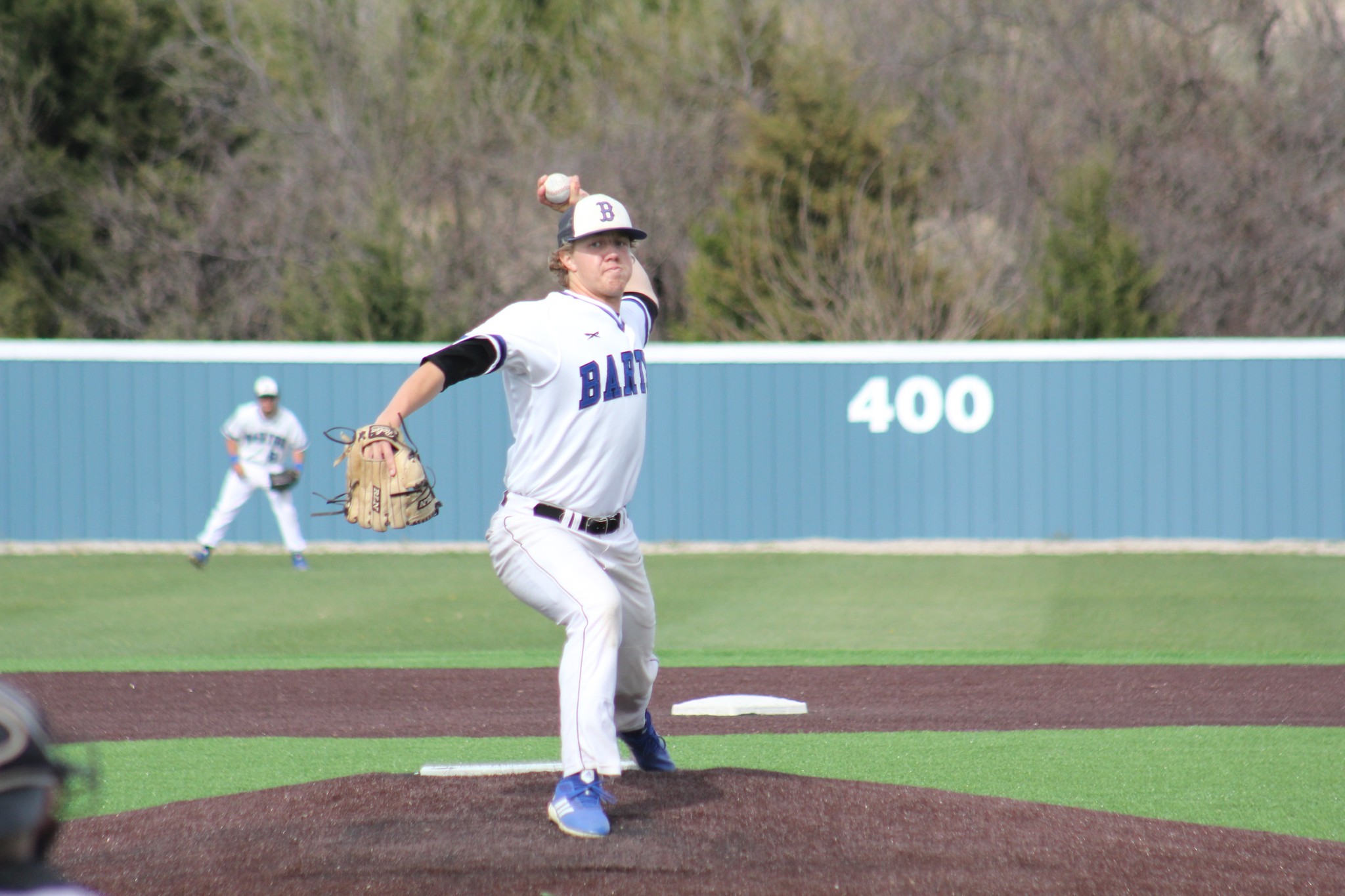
342,424,442,533
269,470,297,491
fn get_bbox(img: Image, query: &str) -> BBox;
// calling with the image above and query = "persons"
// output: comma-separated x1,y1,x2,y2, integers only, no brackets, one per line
363,174,677,839
185,375,310,573
0,684,96,896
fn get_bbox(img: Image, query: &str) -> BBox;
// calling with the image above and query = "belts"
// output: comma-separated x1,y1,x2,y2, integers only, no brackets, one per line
533,503,621,535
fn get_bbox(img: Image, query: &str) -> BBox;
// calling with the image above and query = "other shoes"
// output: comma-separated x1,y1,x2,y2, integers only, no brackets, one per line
292,553,309,572
188,545,211,569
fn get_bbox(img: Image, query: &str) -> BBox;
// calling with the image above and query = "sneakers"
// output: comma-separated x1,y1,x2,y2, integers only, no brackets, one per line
616,708,678,774
547,769,619,839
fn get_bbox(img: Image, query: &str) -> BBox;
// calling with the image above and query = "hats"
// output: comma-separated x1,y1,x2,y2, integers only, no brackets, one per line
557,193,648,248
254,375,280,398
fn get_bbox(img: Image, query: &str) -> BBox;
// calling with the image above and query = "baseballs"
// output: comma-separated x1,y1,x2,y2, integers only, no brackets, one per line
544,172,574,206
